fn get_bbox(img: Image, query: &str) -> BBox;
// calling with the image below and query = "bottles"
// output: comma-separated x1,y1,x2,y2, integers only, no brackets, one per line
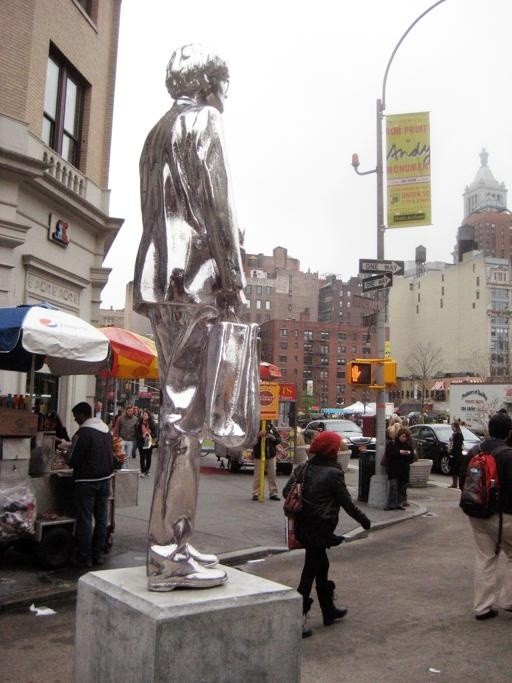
489,479,495,488
8,394,30,409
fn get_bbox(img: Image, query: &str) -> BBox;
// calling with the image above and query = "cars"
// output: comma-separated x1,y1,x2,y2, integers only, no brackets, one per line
110,404,158,431
404,420,483,477
298,415,376,457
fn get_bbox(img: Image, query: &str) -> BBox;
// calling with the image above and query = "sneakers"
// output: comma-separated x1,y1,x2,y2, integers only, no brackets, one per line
140,471,151,477
252,494,258,501
474,605,501,620
65,549,106,569
448,484,457,488
269,495,281,501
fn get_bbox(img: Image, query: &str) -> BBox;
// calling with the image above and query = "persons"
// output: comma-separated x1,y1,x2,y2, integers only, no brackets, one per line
31,398,158,477
131,41,255,597
65,402,115,569
448,423,464,489
282,431,371,638
381,414,418,512
458,415,511,621
496,409,512,446
250,420,281,503
320,412,417,427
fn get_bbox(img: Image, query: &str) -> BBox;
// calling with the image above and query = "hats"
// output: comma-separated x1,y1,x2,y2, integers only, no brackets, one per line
309,432,341,457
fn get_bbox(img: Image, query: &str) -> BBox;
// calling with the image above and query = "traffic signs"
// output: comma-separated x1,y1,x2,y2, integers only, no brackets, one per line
358,258,406,276
362,272,392,293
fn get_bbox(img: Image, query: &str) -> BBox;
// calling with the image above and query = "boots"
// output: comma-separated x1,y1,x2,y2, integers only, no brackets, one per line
302,597,314,638
315,580,348,626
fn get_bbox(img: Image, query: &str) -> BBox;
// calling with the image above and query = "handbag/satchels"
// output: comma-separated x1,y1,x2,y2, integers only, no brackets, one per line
281,458,311,550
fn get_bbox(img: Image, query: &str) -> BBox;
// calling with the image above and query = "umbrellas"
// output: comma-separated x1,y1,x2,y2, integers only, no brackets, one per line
1,302,113,411
97,325,159,382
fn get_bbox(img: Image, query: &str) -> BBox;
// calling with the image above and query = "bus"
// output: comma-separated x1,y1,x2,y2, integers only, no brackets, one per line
135,391,159,411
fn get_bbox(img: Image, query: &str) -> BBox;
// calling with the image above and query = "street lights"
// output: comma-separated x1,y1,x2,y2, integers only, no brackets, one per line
347,149,398,513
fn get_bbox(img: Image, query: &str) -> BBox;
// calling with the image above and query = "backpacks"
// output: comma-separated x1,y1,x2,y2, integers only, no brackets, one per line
457,443,511,519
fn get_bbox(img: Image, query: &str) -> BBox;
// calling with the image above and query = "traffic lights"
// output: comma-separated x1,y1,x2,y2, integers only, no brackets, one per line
384,361,396,384
347,362,371,385
